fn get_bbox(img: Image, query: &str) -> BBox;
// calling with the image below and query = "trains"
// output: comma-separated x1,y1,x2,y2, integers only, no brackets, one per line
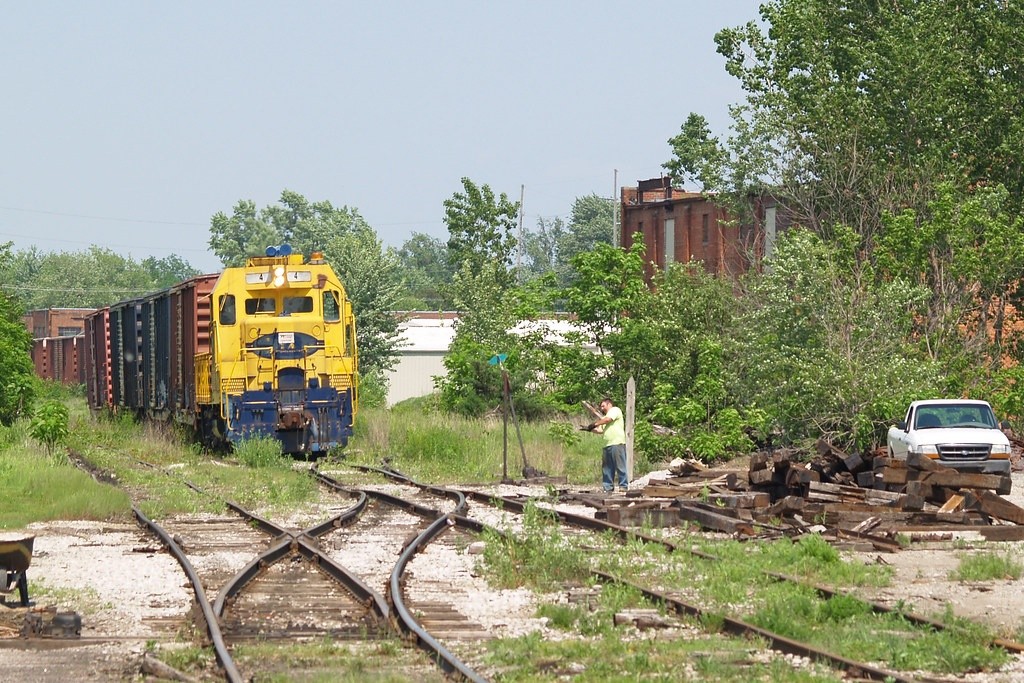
82,243,358,463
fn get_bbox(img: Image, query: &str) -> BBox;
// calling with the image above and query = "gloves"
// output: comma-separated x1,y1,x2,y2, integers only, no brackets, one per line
579,422,596,432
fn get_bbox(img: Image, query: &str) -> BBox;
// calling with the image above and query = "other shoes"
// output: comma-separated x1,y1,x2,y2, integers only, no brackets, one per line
619,487,628,492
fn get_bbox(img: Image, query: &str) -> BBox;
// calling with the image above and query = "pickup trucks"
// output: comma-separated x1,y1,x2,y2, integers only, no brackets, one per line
888,398,1013,496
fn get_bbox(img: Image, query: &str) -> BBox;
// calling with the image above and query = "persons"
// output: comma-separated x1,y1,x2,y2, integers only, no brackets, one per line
579,398,628,492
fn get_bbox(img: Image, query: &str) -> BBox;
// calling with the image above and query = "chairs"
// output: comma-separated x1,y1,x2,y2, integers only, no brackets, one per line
960,415,976,422
918,413,942,426
